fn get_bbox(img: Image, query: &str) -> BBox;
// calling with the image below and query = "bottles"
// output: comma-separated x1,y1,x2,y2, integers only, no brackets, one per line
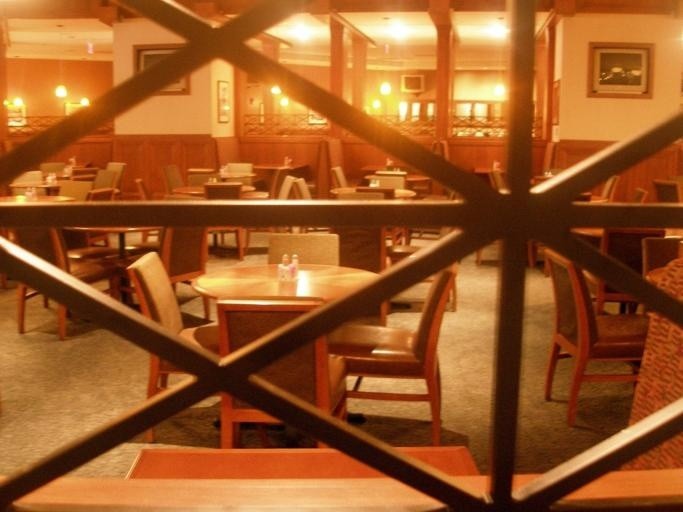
25,187,37,205
280,253,298,279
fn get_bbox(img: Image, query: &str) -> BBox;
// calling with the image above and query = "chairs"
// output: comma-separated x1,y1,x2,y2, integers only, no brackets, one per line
123,250,223,445
541,247,649,427
8,140,468,344
477,141,683,317
216,298,352,449
324,259,461,447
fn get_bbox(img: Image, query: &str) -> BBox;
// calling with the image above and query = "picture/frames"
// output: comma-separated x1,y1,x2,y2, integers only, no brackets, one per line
215,80,232,125
